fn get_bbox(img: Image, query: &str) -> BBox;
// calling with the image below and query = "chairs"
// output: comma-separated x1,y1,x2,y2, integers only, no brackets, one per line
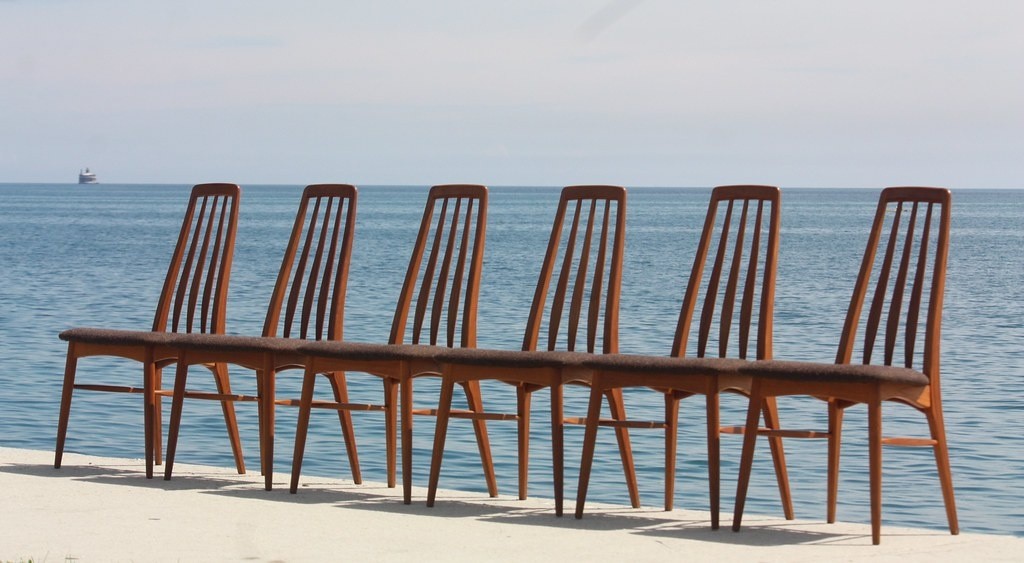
571,183,792,529
289,184,497,506
159,181,362,493
423,186,639,509
50,185,246,478
730,185,960,545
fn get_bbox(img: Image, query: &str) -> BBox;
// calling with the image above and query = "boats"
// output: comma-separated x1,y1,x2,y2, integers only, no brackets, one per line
79,166,99,185
